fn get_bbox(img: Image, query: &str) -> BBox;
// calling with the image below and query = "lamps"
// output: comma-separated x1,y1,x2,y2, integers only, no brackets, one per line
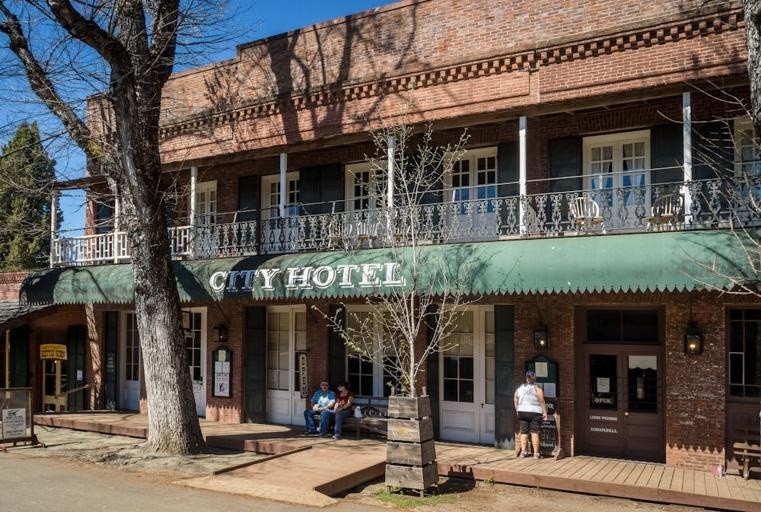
682,292,706,359
211,302,229,343
530,295,550,350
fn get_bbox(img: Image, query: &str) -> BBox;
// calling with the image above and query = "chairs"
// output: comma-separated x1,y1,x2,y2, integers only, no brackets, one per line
732,424,760,480
640,193,685,232
568,197,607,235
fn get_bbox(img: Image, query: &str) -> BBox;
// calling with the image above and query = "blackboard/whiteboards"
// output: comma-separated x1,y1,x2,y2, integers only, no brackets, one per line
528,420,558,457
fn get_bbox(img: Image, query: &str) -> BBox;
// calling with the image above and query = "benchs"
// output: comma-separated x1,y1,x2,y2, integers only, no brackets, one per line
324,233,377,248
311,396,388,441
391,228,445,245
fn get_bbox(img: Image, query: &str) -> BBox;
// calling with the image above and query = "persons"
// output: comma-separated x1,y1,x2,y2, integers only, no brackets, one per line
513,371,547,459
319,381,354,439
304,380,336,436
